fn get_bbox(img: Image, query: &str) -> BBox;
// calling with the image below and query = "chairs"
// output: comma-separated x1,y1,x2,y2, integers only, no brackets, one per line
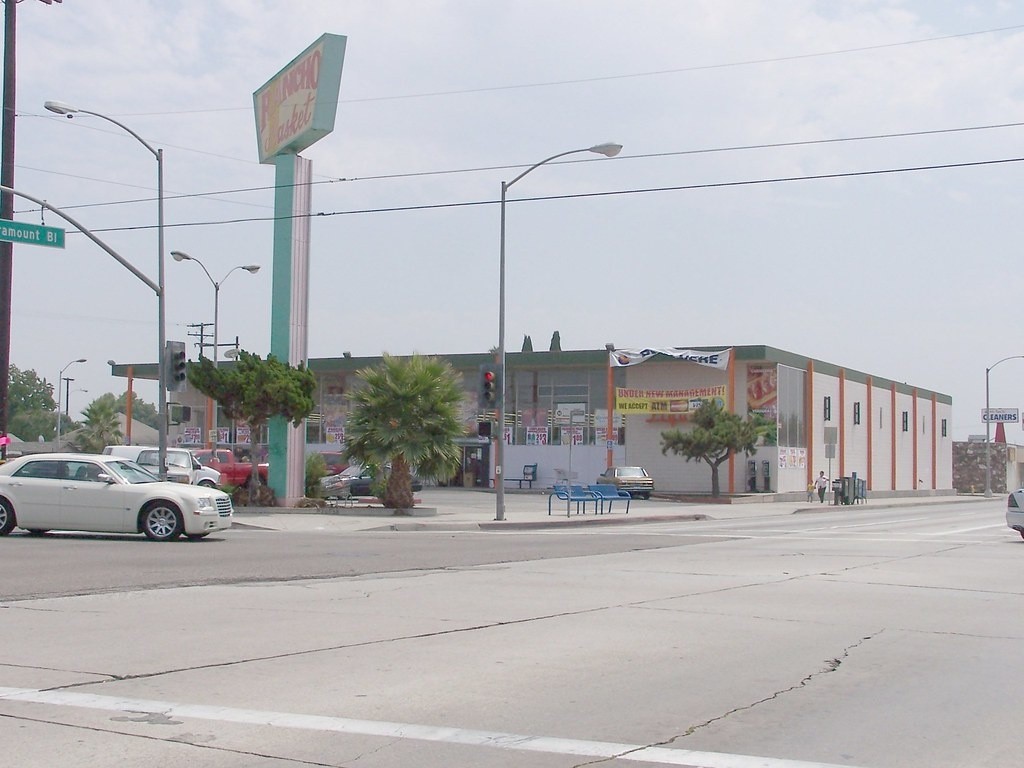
72,465,87,481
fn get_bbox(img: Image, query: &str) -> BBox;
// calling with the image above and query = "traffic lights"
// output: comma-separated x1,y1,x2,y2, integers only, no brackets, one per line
166,341,187,392
479,365,501,407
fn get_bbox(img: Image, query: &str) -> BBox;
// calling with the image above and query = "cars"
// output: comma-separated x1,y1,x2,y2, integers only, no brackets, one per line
1005,489,1024,540
317,463,423,500
595,466,655,500
135,447,203,484
1,453,235,540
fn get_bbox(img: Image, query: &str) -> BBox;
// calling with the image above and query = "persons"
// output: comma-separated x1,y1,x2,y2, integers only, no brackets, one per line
814,471,829,503
806,480,816,502
85,465,103,481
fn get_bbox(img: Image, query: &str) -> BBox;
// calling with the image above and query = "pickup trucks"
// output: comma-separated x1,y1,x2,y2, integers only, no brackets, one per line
193,449,268,488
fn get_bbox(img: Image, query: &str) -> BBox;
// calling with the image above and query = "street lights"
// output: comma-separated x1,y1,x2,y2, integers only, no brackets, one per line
57,358,88,439
496,142,623,518
1,101,168,480
171,250,262,442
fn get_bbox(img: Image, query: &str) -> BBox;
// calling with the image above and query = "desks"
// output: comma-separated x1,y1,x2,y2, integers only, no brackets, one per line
489,479,533,489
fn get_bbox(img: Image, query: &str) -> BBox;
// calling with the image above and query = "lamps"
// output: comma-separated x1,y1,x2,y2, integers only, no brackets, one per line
107,359,115,365
343,351,353,360
605,343,615,351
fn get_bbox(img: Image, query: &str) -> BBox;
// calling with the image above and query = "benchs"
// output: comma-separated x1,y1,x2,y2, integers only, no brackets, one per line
549,485,631,515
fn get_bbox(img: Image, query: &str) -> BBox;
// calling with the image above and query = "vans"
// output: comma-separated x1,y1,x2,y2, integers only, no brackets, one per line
101,446,222,490
316,451,352,477
